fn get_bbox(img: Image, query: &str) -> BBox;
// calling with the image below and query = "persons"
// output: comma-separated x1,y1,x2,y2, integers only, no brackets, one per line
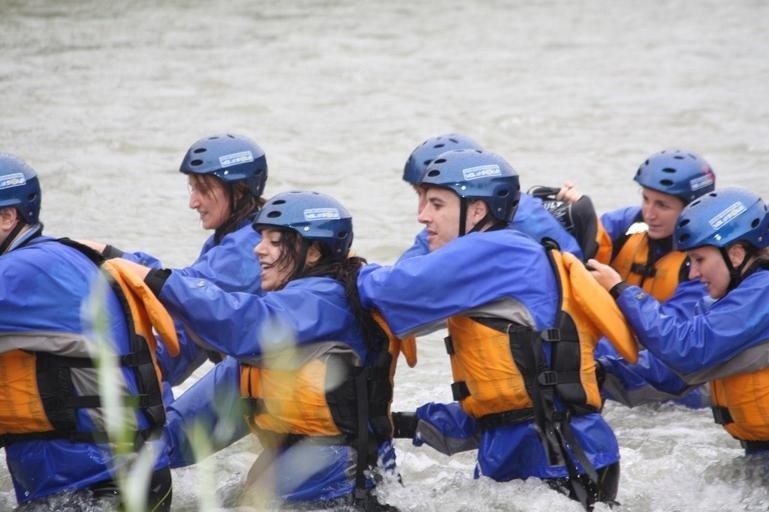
396,132,587,452
115,185,421,511
0,152,171,511
71,133,273,512
561,144,719,413
586,185,768,458
357,148,639,511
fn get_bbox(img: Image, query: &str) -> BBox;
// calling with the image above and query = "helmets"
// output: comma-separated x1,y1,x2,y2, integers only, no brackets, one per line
672,188,768,252
179,136,266,197
253,190,353,256
402,134,520,222
634,148,715,200
0,154,41,222
